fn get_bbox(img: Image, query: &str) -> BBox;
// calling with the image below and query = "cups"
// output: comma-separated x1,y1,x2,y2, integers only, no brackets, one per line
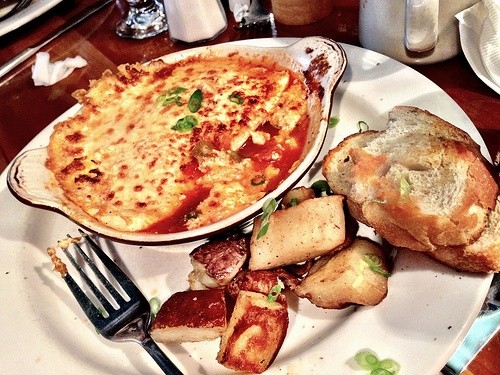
359,0,480,64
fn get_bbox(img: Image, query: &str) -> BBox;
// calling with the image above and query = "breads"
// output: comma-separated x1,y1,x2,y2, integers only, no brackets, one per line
321,130,500,274
347,105,500,252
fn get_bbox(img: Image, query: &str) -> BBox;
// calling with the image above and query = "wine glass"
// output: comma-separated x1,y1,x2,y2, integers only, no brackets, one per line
115,0,168,38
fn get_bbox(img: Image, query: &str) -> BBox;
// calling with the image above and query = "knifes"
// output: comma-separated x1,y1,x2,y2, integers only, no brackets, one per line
0,0,114,78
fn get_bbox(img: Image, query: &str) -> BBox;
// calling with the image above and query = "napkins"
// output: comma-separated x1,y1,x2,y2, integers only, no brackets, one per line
455,0,500,96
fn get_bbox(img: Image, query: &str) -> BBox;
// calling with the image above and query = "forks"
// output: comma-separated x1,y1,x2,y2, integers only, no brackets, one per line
47,229,183,375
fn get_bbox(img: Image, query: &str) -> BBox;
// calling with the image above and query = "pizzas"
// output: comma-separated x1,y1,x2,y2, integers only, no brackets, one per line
43,53,322,236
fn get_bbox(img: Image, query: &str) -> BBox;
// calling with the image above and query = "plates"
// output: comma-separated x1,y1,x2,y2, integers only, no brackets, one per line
0,0,63,36
6,35,348,246
0,38,495,375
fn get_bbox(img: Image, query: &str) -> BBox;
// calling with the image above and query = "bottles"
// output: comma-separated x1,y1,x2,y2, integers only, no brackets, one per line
163,0,228,44
